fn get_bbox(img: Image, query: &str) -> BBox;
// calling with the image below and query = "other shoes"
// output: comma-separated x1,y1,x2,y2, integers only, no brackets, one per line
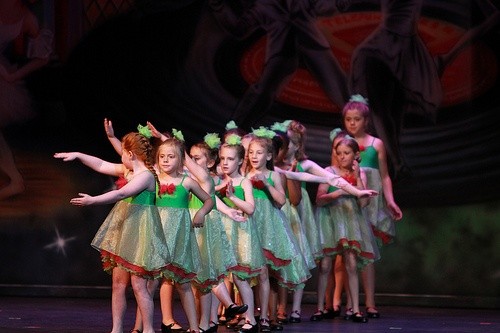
129,329,142,333
187,312,289,333
290,310,301,323
161,322,187,333
310,310,324,321
334,305,341,316
352,313,369,323
366,307,380,319
323,310,332,318
225,304,248,322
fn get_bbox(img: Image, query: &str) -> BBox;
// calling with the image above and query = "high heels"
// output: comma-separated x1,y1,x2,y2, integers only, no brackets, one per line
343,308,353,320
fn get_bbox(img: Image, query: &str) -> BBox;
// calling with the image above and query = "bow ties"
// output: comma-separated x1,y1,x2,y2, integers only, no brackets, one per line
115,172,128,189
220,186,234,196
359,145,366,151
251,179,264,188
160,183,176,194
342,174,357,186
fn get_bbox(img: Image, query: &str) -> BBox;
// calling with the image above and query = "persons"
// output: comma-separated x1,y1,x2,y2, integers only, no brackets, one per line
53,118,378,333
211,0,349,128
332,94,403,319
351,0,500,188
0,0,58,200
